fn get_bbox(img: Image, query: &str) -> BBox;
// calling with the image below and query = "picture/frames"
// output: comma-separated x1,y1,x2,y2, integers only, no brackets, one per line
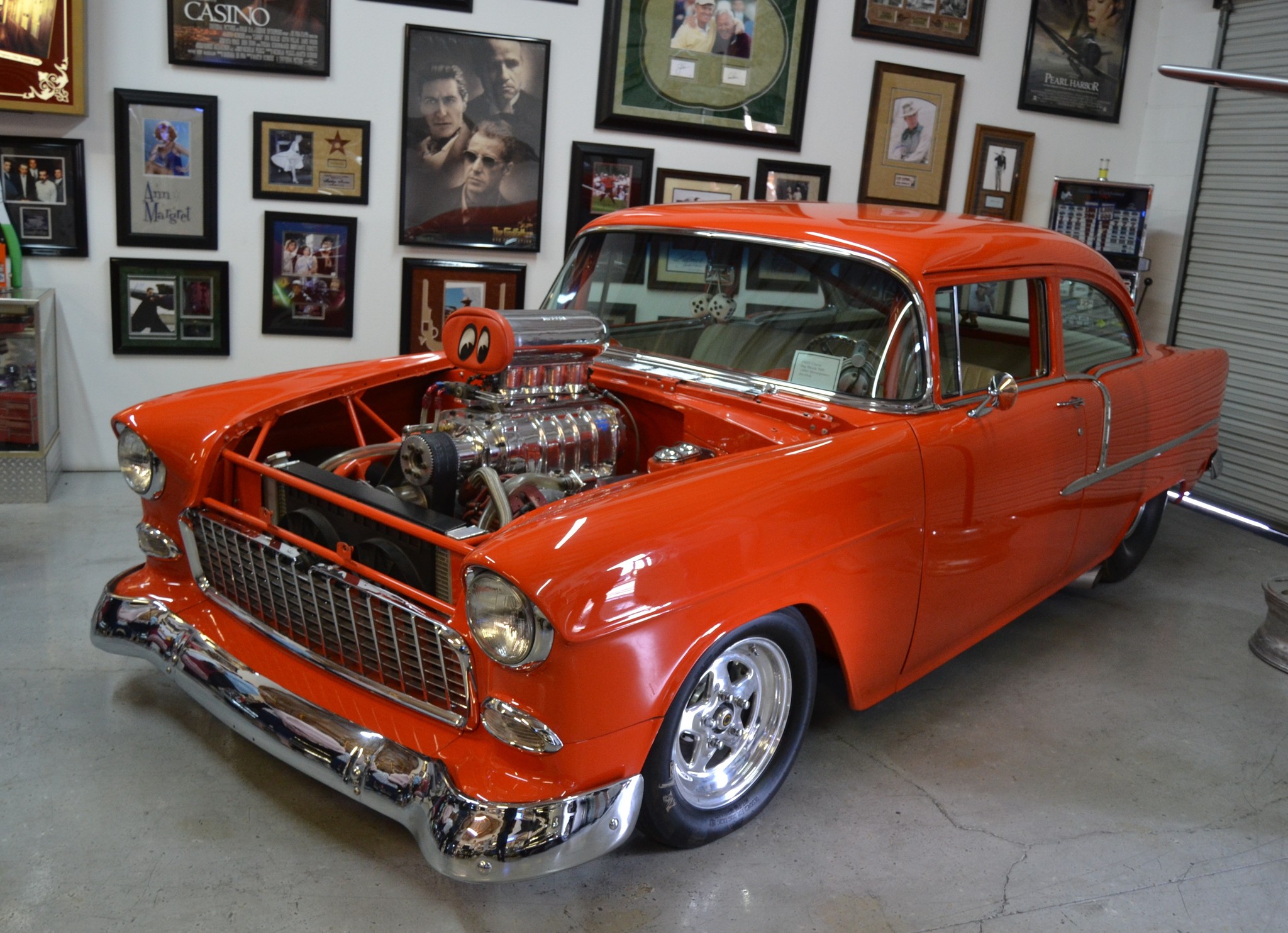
107,256,232,357
746,157,831,297
114,86,218,248
250,110,372,208
398,254,529,356
593,0,823,154
398,23,551,252
850,0,988,58
563,139,658,288
0,0,87,117
644,165,748,287
585,299,638,328
963,124,1038,223
1014,0,1138,123
856,59,966,213
260,209,360,340
165,0,333,80
1,133,92,261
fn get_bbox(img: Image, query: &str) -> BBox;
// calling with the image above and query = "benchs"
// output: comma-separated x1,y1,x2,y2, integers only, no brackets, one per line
688,322,1006,418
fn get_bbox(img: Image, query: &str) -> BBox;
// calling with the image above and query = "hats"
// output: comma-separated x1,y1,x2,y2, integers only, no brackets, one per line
694,0,717,7
898,100,923,120
460,296,473,303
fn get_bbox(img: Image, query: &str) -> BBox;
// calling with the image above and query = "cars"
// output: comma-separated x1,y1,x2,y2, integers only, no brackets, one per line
82,194,1232,890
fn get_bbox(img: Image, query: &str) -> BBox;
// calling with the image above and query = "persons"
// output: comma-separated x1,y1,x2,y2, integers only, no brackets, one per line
275,134,305,183
993,149,1008,190
3,160,16,199
28,158,39,181
897,100,931,163
54,168,64,202
1086,0,1126,47
671,1,754,60
145,120,189,176
35,170,58,202
792,187,803,201
784,186,793,200
13,163,36,200
130,287,174,334
408,36,544,224
188,279,211,316
461,296,472,307
278,228,347,324
593,162,631,214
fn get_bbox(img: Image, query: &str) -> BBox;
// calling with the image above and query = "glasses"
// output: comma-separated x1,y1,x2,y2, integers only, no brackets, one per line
462,149,506,169
